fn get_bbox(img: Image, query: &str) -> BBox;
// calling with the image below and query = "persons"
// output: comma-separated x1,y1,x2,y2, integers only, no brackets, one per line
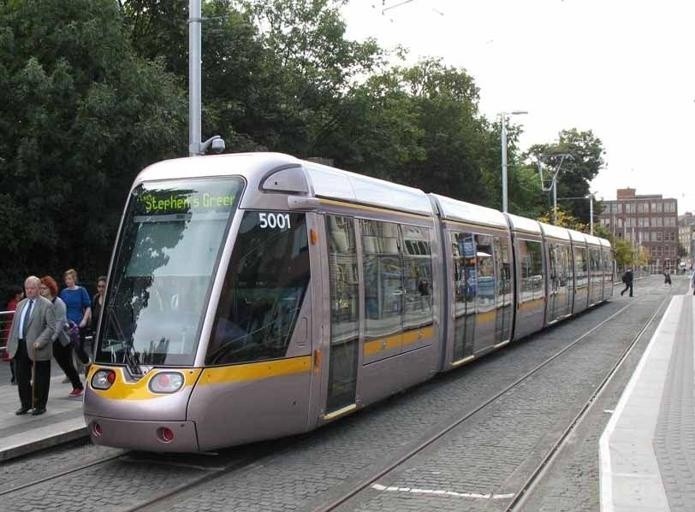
5,274,56,417
38,276,86,399
89,275,107,340
620,267,634,298
418,277,433,312
60,267,94,383
3,284,26,385
663,267,673,285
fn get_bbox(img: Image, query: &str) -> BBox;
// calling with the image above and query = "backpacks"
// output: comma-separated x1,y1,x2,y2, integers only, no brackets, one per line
622,272,630,282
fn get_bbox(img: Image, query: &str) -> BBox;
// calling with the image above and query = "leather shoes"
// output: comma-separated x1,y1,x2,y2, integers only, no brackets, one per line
32,407,46,416
16,407,30,415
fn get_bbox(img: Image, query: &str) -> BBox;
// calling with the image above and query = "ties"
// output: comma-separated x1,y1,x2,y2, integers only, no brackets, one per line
23,299,34,342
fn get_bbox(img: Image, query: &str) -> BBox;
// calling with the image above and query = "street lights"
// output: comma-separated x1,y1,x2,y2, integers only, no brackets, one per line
589,190,599,235
498,108,534,213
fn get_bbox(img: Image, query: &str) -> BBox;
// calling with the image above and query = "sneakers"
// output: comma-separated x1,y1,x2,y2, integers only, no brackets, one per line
69,385,84,396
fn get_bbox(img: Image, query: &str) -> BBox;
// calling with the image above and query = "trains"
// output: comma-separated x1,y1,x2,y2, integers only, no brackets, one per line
82,148,616,461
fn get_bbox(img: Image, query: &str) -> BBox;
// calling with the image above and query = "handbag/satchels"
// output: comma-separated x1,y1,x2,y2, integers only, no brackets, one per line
66,319,81,348
